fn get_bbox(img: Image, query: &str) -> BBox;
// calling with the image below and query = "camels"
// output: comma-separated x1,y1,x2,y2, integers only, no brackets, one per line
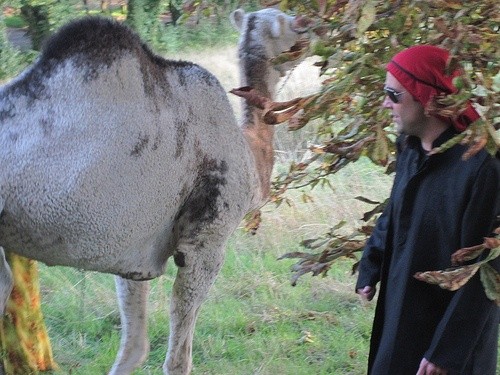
0,9,327,375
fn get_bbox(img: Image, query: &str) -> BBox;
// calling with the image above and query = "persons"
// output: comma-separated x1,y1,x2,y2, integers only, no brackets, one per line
355,45,500,375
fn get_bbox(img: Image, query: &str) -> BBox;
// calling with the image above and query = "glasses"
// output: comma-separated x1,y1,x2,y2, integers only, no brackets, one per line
384,87,407,103
